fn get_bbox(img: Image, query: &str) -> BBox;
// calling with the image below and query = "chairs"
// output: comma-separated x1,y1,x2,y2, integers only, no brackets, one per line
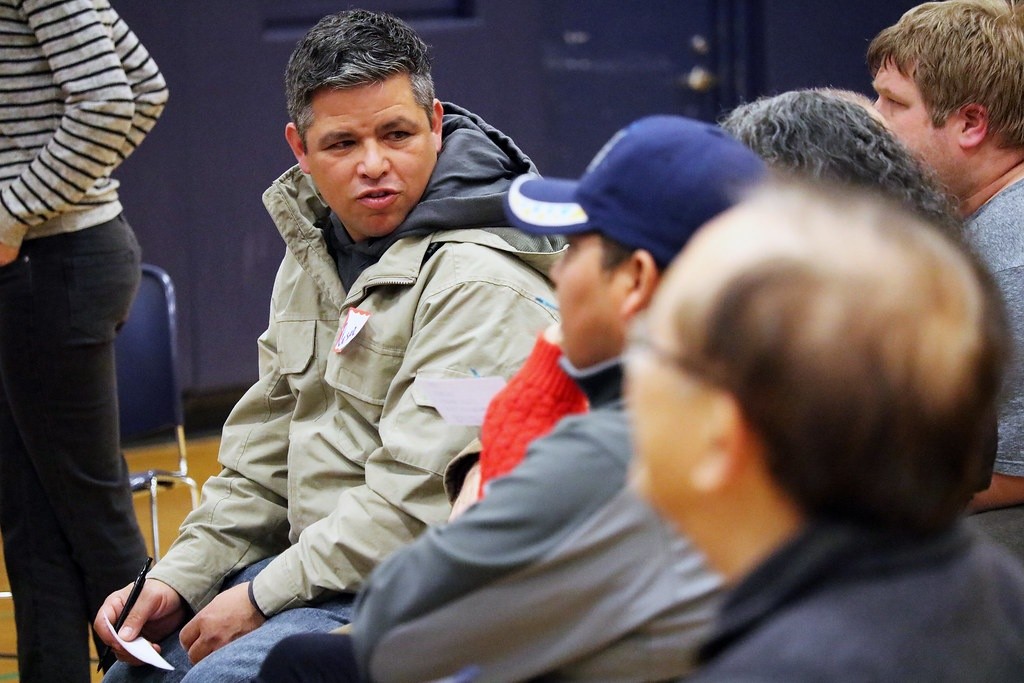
116,266,200,566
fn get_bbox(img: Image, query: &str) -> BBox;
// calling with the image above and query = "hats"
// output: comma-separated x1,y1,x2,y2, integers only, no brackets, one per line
503,111,769,266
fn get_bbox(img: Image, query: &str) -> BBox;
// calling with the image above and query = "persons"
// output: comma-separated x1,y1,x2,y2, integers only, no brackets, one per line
620,185,1024,683
256,89,948,683
93,8,570,683
0,0,169,683
865,0,1024,519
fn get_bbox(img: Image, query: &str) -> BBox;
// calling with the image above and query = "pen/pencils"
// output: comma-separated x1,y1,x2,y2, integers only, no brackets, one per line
96,557,153,673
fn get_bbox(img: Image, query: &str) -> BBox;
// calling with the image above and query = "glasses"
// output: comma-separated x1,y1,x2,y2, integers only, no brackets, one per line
620,312,741,402
